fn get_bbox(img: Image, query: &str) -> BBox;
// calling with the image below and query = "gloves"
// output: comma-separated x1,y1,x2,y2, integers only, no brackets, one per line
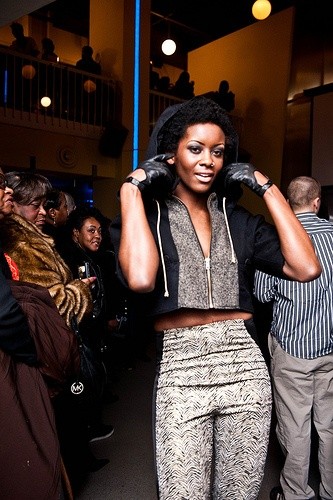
223,161,273,199
121,152,176,195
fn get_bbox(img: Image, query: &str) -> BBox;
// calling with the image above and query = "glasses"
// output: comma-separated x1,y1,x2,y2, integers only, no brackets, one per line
0,173,7,191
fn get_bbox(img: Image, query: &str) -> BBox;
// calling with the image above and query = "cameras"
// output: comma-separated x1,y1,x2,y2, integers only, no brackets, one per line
78,262,90,279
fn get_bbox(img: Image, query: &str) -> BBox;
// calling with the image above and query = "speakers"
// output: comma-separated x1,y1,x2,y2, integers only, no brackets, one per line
99,120,129,159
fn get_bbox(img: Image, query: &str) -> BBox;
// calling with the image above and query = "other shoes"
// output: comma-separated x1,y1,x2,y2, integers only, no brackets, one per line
84,423,114,443
84,456,109,473
269,486,285,500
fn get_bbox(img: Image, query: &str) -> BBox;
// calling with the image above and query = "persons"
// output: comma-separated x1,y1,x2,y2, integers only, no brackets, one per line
117,96,322,500
150,71,234,113
7,22,103,125
0,166,61,500
252,176,333,500
43,189,118,442
5,170,99,472
